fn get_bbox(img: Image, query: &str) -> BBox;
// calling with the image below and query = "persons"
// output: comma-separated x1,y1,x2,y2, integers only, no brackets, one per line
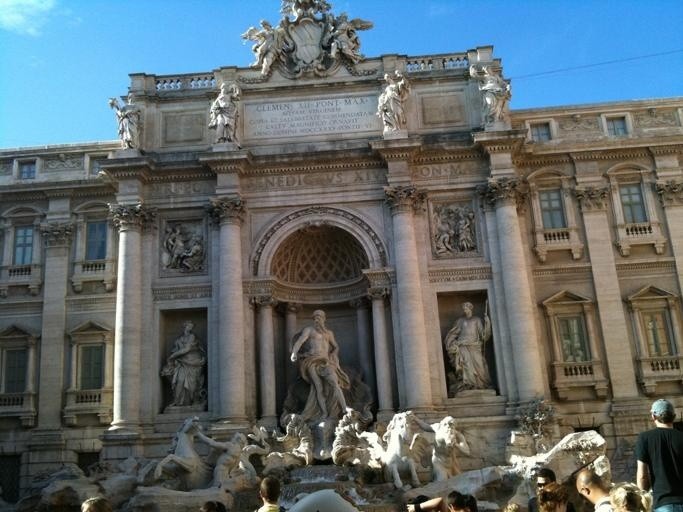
633,398,683,512
159,318,207,406
288,310,351,419
403,466,654,511
374,68,413,133
196,430,247,488
319,10,374,61
442,302,493,392
80,495,112,512
107,91,146,150
432,208,475,258
206,82,246,149
405,408,470,481
248,476,287,512
166,223,205,273
468,62,511,123
238,19,295,80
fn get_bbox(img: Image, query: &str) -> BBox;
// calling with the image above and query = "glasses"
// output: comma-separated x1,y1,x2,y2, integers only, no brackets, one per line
537,482,548,488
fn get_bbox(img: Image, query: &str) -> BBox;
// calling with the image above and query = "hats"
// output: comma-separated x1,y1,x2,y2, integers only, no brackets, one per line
649,397,675,416
